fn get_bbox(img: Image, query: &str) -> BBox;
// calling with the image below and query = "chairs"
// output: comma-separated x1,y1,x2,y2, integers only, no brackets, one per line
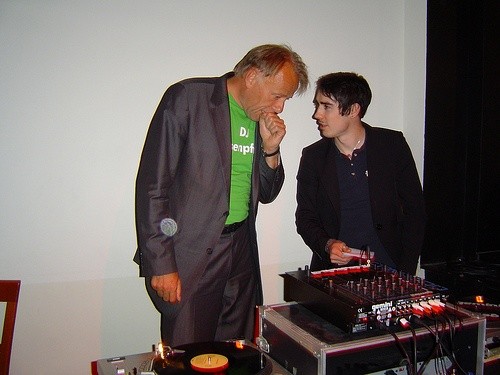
0,280,21,375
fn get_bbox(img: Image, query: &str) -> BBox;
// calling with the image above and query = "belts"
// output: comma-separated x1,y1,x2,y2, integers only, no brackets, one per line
222,214,250,234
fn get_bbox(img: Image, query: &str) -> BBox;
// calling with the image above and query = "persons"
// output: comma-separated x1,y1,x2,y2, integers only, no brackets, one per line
291,72,426,280
133,43,312,357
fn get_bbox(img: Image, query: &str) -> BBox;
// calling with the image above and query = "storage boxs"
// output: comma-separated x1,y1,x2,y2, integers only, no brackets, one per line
256,300,487,375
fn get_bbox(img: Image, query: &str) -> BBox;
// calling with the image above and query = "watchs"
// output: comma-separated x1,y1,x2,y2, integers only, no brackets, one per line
260,144,280,158
